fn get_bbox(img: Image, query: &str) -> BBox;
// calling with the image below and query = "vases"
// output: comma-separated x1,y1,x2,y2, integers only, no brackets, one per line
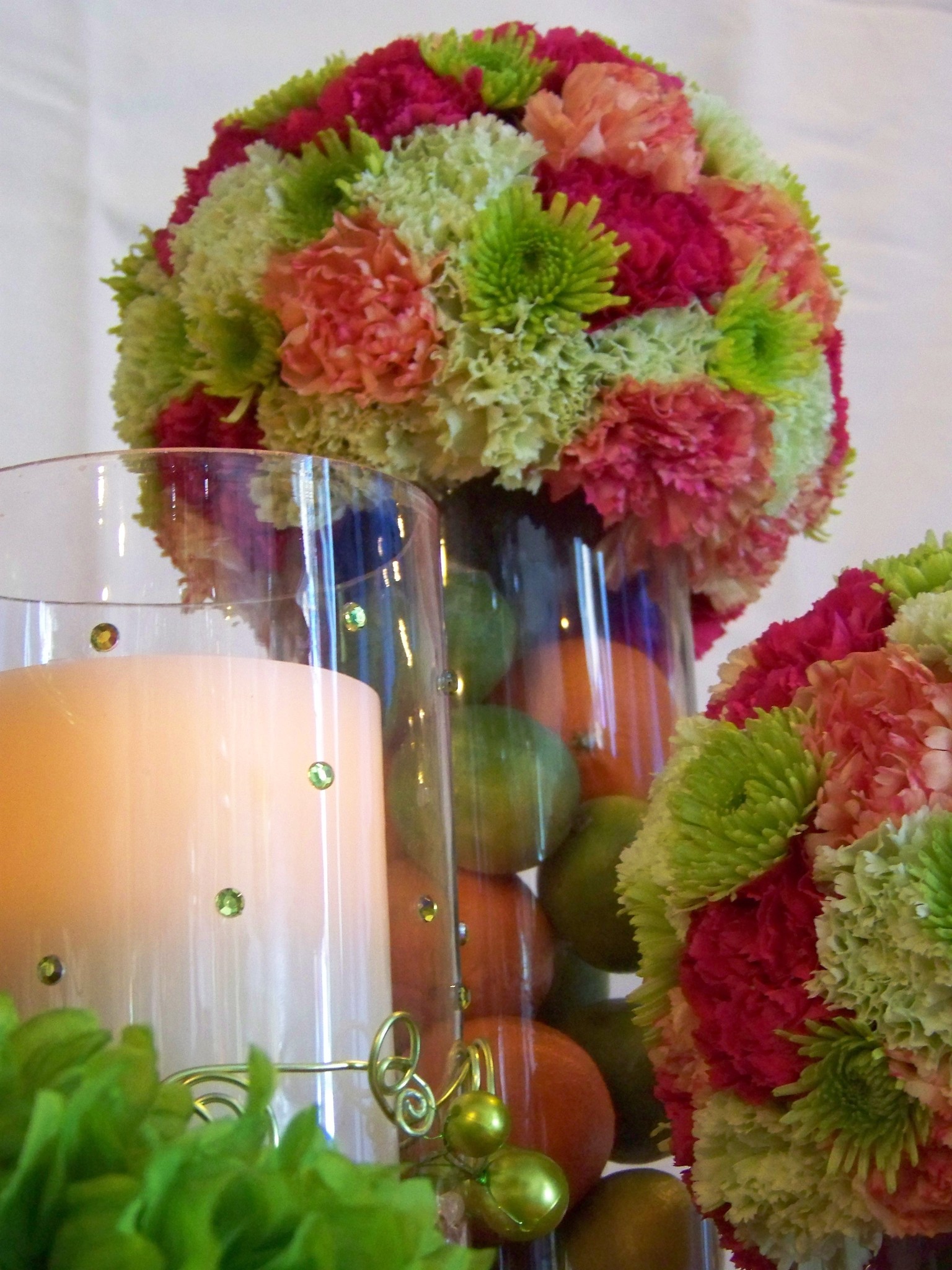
416,461,736,1269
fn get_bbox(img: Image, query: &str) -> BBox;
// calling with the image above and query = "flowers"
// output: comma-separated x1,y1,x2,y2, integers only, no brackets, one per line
102,8,862,658
7,990,503,1270
612,540,952,1270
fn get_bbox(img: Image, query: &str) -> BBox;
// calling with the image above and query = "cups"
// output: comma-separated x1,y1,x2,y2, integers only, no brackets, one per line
1,445,477,1257
248,474,740,1268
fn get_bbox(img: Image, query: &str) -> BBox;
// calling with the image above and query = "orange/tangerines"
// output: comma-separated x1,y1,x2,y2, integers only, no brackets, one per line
334,567,702,1269
